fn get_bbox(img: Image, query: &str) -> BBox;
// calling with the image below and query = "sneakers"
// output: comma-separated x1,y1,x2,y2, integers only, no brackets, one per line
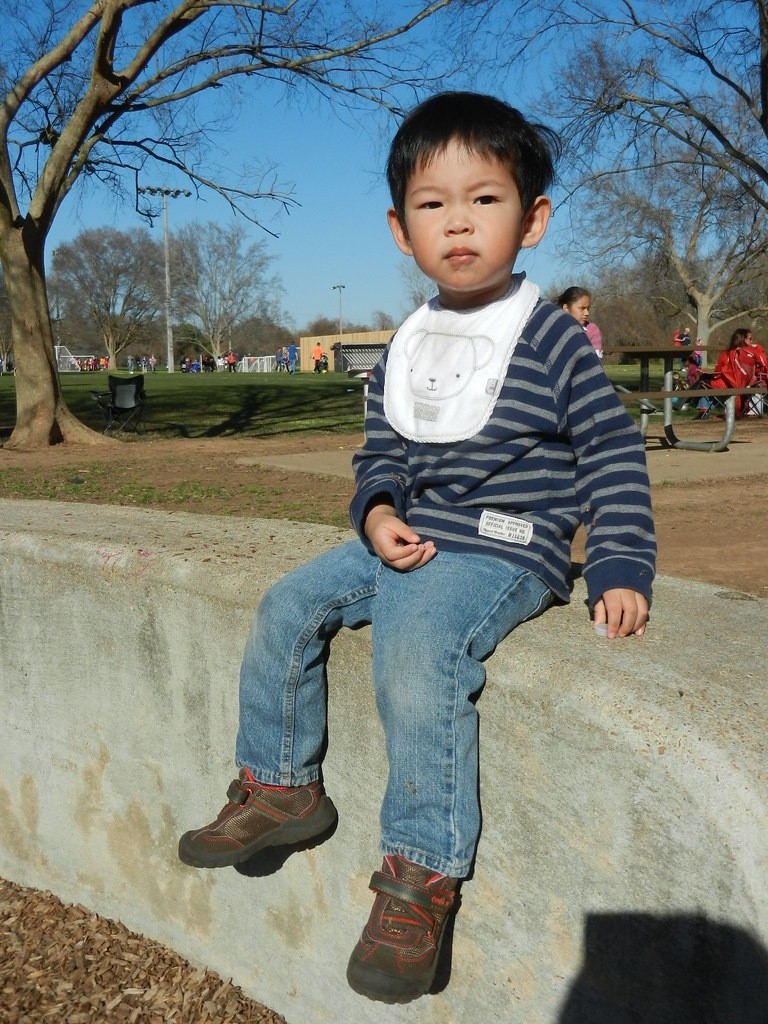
347,852,459,1005
178,773,337,870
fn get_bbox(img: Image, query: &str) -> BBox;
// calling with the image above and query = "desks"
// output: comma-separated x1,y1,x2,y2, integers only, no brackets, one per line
603,343,730,441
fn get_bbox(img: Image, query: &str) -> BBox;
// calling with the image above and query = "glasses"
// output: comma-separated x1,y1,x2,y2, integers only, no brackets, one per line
746,337,752,342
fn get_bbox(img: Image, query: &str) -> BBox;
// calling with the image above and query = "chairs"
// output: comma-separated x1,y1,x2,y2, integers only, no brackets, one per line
695,347,768,421
89,374,148,436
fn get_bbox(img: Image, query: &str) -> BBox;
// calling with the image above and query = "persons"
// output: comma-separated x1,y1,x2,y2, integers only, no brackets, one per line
185,350,236,373
275,341,323,376
557,286,604,364
0,359,13,376
128,353,157,374
69,355,110,371
675,327,768,420
178,91,655,1003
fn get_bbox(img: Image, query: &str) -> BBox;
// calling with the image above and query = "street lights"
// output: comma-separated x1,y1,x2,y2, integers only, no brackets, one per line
330,284,346,373
135,184,191,374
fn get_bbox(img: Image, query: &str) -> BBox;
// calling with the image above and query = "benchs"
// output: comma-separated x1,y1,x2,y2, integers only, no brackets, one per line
616,388,768,452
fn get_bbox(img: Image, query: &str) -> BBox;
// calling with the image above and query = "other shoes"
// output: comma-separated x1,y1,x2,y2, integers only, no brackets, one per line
692,412,709,419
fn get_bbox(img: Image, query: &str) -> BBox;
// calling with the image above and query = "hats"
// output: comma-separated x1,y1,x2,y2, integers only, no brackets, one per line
685,328,689,332
